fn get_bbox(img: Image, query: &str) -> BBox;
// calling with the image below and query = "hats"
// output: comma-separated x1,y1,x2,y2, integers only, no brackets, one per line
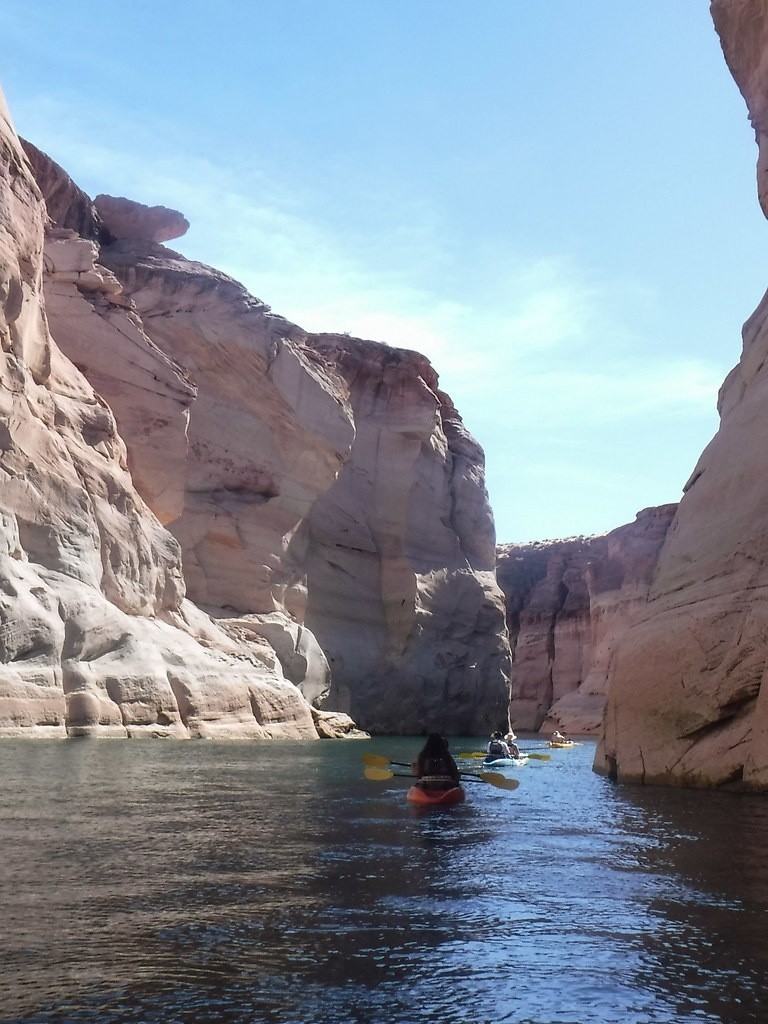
504,732,518,741
491,731,503,739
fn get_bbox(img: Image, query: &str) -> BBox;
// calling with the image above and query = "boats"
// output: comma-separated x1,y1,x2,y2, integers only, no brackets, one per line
481,751,529,769
406,778,465,811
548,739,573,749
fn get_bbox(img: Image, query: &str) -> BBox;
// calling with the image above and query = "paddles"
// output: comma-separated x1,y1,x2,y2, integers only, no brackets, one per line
362,752,506,781
458,751,551,762
363,766,520,792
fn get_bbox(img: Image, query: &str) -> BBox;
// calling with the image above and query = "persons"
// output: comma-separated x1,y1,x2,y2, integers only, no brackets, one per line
418,733,461,791
504,733,519,759
485,731,510,762
551,731,566,743
